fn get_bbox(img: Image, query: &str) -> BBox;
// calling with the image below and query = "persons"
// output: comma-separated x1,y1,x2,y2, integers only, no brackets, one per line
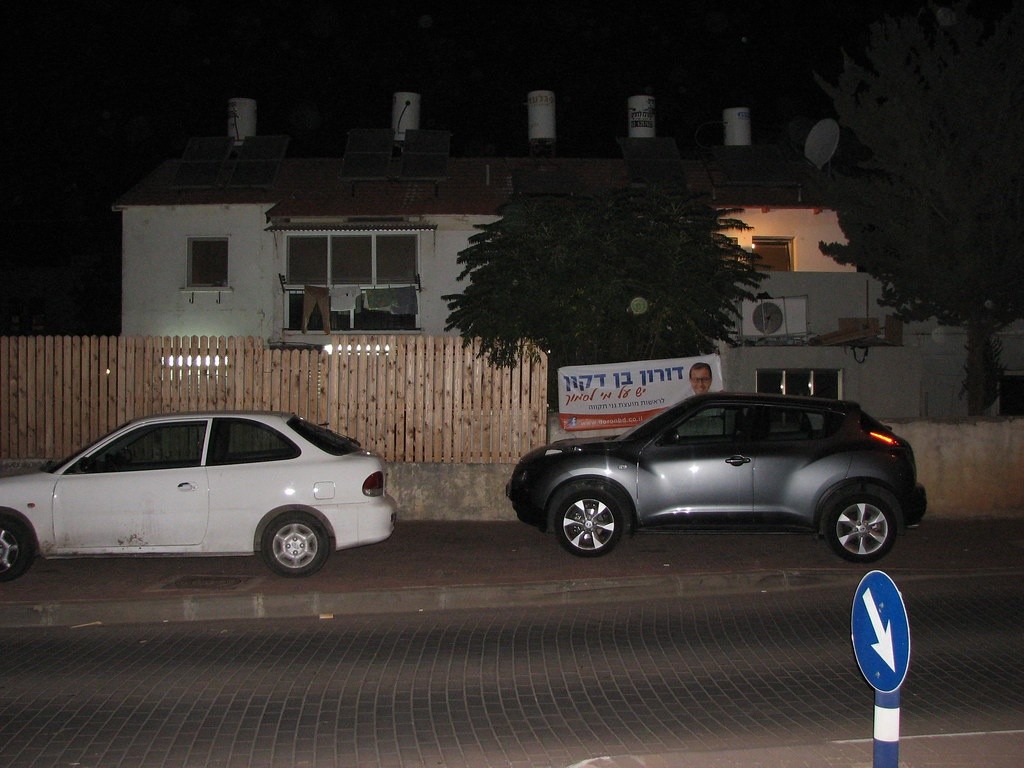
689,363,712,395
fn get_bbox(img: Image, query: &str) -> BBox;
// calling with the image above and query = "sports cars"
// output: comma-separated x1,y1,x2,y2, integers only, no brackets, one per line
504,395,927,563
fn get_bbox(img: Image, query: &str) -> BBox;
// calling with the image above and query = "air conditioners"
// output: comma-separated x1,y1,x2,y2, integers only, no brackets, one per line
738,297,807,337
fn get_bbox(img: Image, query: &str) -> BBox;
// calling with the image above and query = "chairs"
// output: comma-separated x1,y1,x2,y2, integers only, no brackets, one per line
734,412,746,439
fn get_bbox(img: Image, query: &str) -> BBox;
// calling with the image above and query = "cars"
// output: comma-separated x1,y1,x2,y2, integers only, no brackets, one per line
0,407,399,578
